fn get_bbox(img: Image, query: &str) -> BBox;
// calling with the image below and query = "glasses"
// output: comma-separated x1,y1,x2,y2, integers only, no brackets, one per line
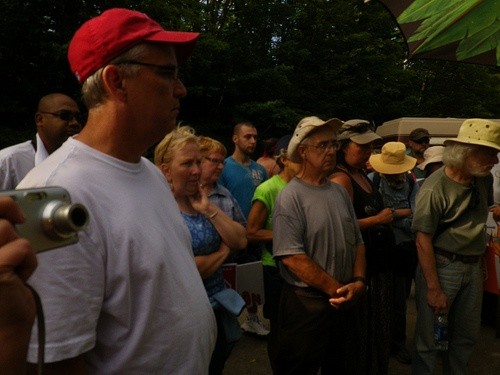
302,142,340,153
115,60,181,79
340,120,375,134
205,157,224,164
40,110,85,124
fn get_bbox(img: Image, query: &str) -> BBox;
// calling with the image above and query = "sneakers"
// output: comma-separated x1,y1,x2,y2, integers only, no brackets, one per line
240,316,270,336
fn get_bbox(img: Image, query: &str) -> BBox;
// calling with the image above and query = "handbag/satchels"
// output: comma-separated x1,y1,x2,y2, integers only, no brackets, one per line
209,288,247,342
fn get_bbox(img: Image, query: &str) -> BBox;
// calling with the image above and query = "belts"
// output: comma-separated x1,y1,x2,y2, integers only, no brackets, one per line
432,246,482,263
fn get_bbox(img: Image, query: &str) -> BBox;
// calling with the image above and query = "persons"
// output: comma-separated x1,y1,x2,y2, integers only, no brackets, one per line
0,93,82,192
15,7,217,375
0,196,39,375
153,117,500,375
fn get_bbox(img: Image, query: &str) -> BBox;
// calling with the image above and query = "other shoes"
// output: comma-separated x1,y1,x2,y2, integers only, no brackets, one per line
390,344,412,363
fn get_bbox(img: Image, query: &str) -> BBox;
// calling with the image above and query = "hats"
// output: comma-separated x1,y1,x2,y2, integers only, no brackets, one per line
67,7,200,85
368,142,417,174
286,116,344,163
443,118,500,153
417,146,445,170
338,119,383,145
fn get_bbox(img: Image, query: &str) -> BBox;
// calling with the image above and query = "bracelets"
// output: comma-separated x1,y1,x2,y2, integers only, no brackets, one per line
207,208,219,218
351,275,370,293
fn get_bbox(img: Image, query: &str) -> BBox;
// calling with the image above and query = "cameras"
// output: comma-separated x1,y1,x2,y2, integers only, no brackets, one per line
1,182,91,255
